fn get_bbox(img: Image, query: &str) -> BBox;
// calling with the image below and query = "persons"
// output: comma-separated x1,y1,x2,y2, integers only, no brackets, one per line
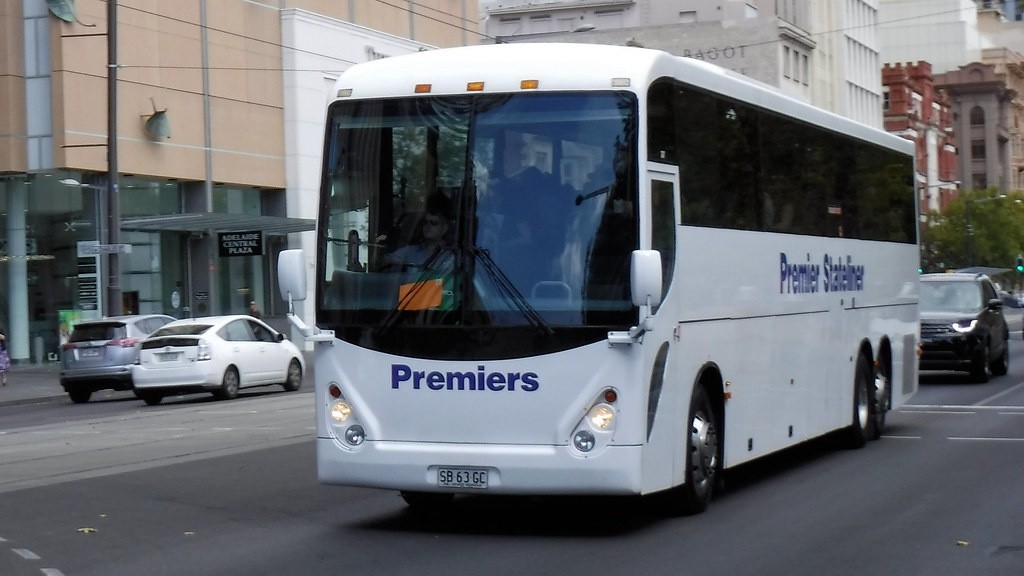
680,159,918,243
0,327,11,386
379,207,455,271
248,300,264,341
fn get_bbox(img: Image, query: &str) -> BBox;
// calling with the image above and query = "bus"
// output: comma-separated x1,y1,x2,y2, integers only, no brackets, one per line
275,39,925,518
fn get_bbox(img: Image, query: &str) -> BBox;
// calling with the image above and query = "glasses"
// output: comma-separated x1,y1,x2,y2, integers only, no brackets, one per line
423,219,445,226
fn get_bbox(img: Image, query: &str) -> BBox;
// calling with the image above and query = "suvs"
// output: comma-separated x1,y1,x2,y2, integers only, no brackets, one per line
56,313,181,401
916,273,1010,385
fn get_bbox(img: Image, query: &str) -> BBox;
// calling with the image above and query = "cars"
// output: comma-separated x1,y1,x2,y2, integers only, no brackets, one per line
131,314,307,405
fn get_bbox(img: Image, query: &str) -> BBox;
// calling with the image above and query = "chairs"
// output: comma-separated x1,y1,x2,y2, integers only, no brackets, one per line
392,185,605,305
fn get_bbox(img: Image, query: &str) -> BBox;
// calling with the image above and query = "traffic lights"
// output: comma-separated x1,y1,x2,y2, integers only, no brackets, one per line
1016,257,1024,273
